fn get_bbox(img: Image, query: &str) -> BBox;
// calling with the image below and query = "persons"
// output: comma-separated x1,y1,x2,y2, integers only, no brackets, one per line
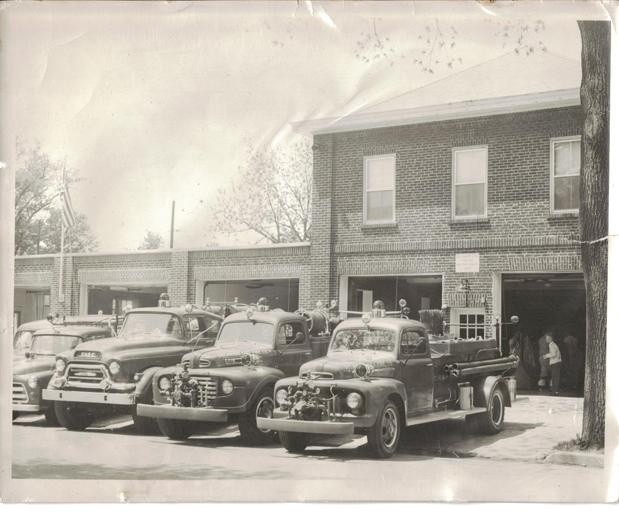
501,325,585,396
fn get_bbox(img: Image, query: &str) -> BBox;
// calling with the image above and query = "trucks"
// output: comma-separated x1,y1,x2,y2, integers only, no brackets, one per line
254,305,522,460
41,290,274,430
12,318,125,428
132,296,351,447
